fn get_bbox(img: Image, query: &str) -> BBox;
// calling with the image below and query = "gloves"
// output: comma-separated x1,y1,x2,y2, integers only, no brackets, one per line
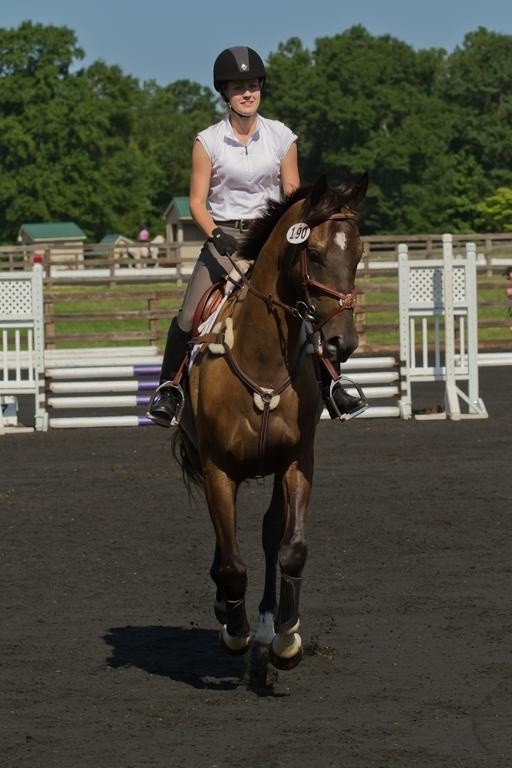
207,229,238,257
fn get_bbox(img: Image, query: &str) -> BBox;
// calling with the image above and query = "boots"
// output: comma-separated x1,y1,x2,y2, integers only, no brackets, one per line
320,359,366,420
150,318,194,419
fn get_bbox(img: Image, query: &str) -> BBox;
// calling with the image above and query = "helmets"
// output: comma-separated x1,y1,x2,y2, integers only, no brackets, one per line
213,46,267,95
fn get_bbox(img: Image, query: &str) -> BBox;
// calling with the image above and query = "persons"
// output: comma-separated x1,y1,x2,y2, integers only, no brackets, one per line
137,224,150,242
501,264,512,302
143,41,366,430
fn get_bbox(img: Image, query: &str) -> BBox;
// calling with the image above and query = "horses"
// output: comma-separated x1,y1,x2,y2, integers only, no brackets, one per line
169,162,370,677
